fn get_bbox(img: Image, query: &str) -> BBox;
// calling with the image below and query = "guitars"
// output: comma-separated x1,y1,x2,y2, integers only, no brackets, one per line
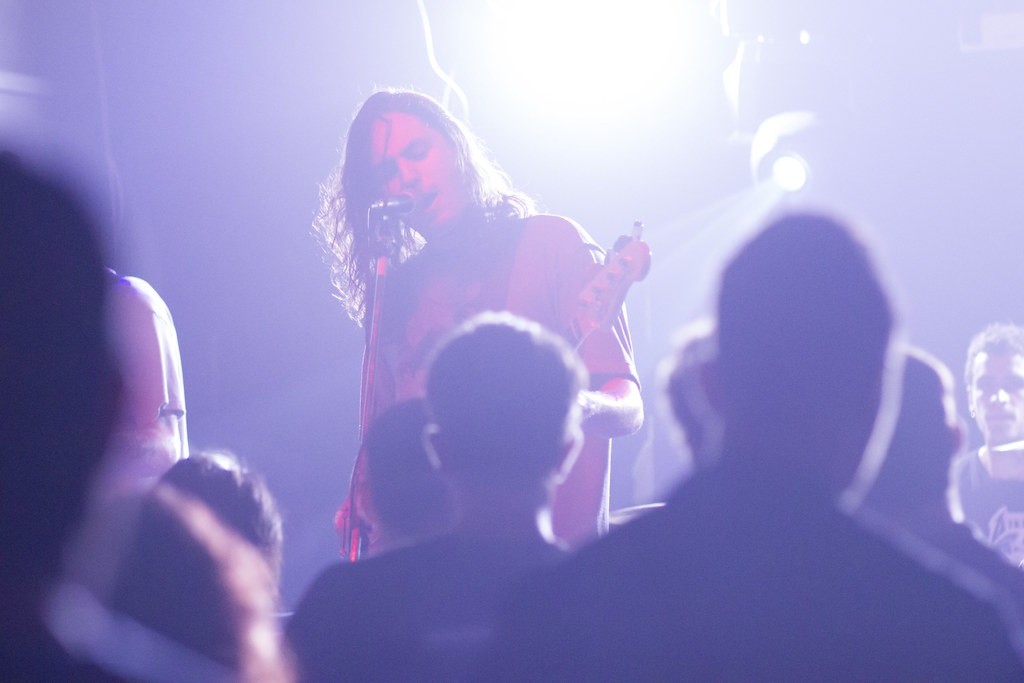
335,219,660,559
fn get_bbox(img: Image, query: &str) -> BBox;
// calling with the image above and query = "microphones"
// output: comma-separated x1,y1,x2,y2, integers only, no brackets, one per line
370,192,414,213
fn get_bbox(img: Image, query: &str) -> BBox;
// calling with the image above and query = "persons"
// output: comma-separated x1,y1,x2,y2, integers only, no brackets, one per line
275,309,589,683
665,326,726,477
848,343,1022,673
317,88,644,558
0,150,298,683
351,393,471,560
949,320,1024,568
479,209,1023,683
